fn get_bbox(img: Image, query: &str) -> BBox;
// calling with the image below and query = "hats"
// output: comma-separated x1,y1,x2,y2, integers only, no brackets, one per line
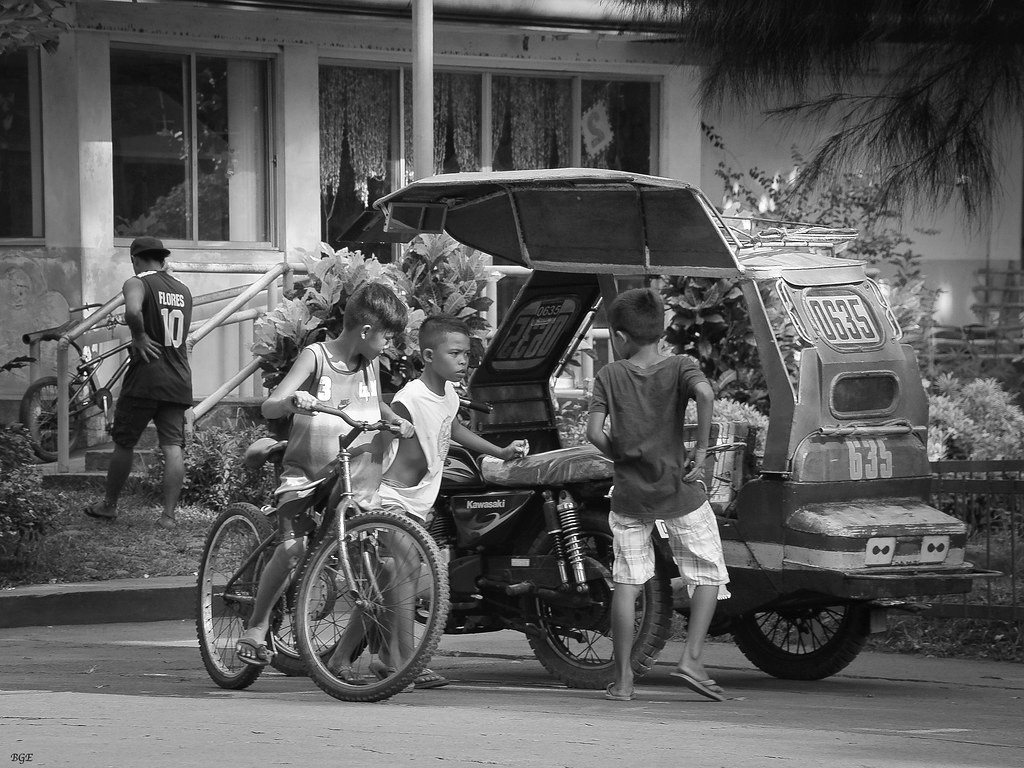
130,236,172,258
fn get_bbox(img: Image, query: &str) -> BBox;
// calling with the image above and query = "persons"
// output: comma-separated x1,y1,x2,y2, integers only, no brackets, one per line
235,282,529,692
84,236,193,529
586,288,730,701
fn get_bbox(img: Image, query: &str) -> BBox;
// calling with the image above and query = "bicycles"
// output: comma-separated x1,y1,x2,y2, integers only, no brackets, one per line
15,302,139,461
193,396,452,702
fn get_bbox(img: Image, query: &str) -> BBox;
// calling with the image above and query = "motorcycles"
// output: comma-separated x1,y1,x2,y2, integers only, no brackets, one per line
345,167,987,700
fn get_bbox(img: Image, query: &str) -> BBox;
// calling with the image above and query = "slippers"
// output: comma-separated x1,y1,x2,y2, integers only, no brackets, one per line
235,637,272,666
325,660,368,685
369,658,415,693
605,682,637,700
85,503,118,520
408,663,450,688
669,667,725,701
153,518,176,532
